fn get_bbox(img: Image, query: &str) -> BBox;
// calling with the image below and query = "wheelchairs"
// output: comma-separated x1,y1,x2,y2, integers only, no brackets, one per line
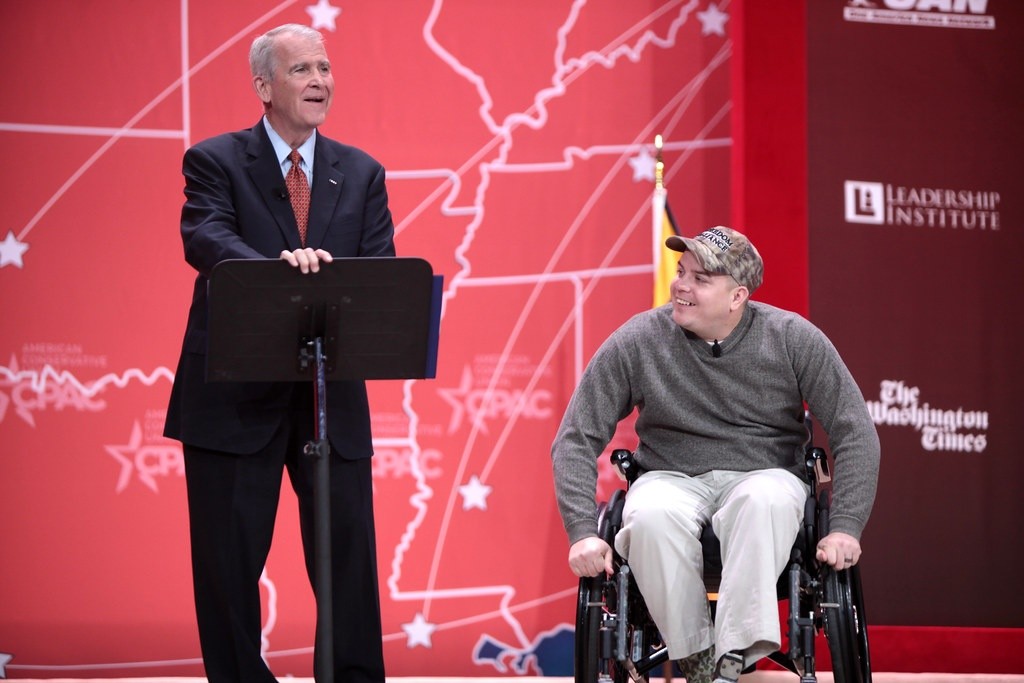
574,447,873,683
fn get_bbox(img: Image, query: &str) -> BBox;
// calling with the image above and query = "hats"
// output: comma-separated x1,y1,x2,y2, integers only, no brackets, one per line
665,226,764,296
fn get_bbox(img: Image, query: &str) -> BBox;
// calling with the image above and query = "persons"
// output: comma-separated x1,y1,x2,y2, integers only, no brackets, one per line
548,224,886,683
161,18,398,681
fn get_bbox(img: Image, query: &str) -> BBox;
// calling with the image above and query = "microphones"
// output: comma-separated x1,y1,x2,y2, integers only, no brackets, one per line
281,191,287,199
712,339,721,358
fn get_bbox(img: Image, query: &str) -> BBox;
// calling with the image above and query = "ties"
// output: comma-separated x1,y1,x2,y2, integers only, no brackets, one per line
285,149,311,250
